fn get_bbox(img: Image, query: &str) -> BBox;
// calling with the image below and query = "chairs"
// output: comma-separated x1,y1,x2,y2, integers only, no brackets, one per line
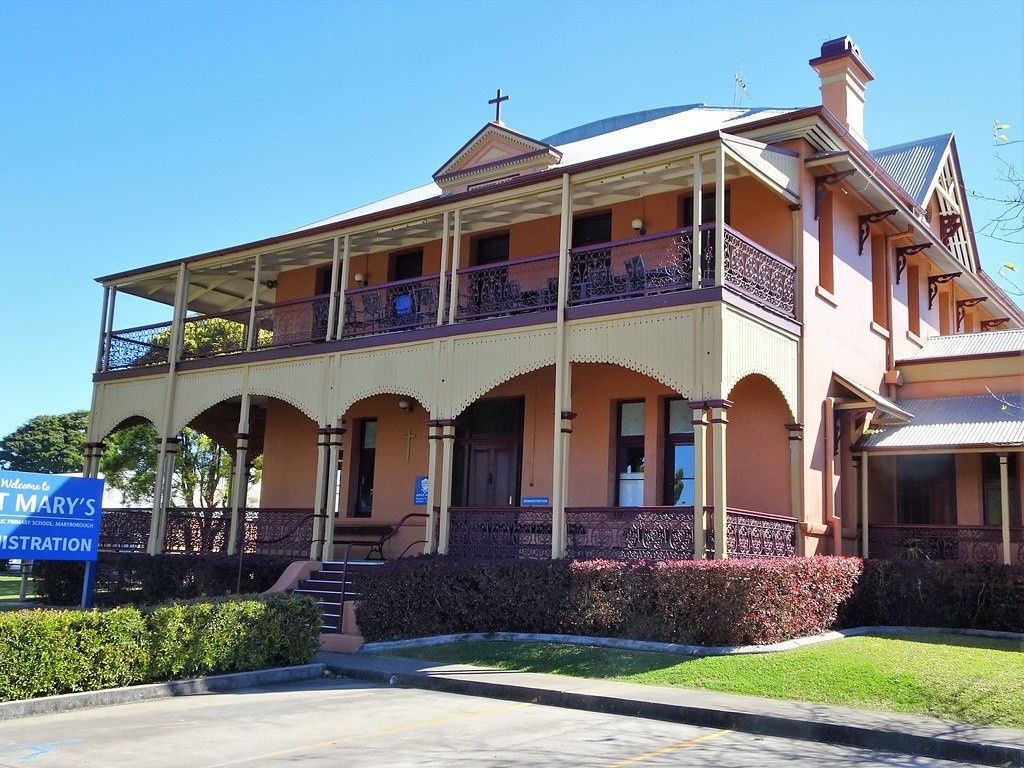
344,294,370,337
546,274,581,308
502,278,532,315
411,283,449,327
479,277,507,318
439,279,479,321
590,266,619,303
388,286,424,331
625,255,668,298
361,292,395,334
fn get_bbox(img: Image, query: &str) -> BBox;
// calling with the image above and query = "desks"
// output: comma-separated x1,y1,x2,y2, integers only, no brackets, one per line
513,266,685,313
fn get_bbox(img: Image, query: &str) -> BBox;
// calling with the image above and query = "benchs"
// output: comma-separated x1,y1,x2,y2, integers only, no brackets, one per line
323,525,392,559
478,523,587,558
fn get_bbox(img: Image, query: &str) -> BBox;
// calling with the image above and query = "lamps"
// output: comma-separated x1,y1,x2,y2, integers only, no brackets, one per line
355,273,369,289
397,401,413,416
631,219,647,236
